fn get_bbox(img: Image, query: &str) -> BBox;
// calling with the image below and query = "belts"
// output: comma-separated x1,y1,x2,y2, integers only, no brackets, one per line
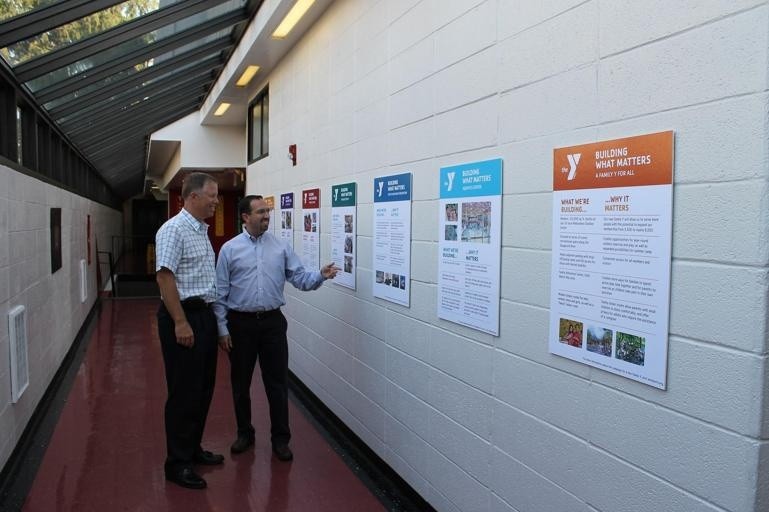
232,310,280,320
183,300,213,309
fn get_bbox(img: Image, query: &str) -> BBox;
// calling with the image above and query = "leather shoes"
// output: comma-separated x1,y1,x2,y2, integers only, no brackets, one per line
272,444,294,462
164,468,207,490
194,451,225,465
230,436,255,454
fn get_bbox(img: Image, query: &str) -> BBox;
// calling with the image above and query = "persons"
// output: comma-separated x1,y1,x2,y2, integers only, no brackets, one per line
445,203,459,222
563,324,581,348
210,193,343,463
153,171,228,490
303,212,312,232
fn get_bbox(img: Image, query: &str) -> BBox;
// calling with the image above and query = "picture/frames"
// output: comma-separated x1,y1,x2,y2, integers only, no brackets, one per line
7,305,29,404
